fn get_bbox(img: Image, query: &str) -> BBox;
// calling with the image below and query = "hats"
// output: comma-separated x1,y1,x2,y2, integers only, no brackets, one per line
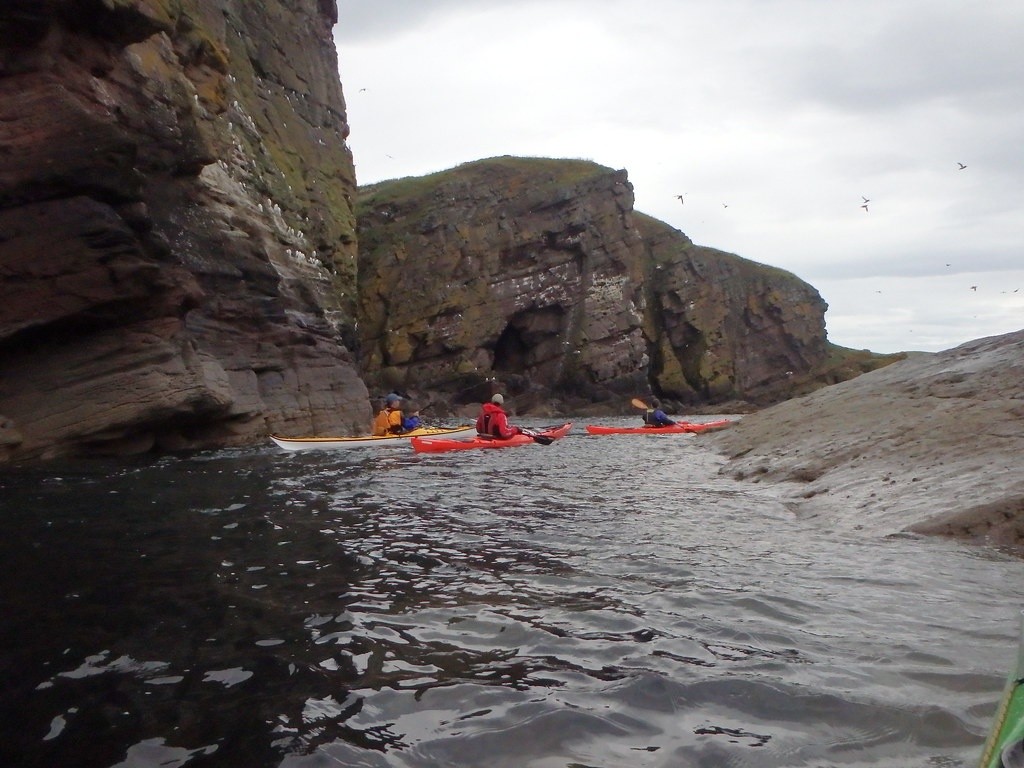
384,394,402,406
492,394,503,404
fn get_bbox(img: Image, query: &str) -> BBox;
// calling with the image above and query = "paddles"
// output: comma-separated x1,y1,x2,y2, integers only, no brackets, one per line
409,401,437,420
632,399,694,432
470,418,555,445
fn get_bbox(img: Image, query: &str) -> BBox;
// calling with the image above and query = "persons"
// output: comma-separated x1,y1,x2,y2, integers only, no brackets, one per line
477,393,523,439
642,399,677,426
373,394,420,434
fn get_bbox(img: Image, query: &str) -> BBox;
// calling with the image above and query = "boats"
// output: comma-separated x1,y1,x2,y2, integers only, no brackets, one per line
586,420,729,434
411,422,572,455
269,424,479,451
